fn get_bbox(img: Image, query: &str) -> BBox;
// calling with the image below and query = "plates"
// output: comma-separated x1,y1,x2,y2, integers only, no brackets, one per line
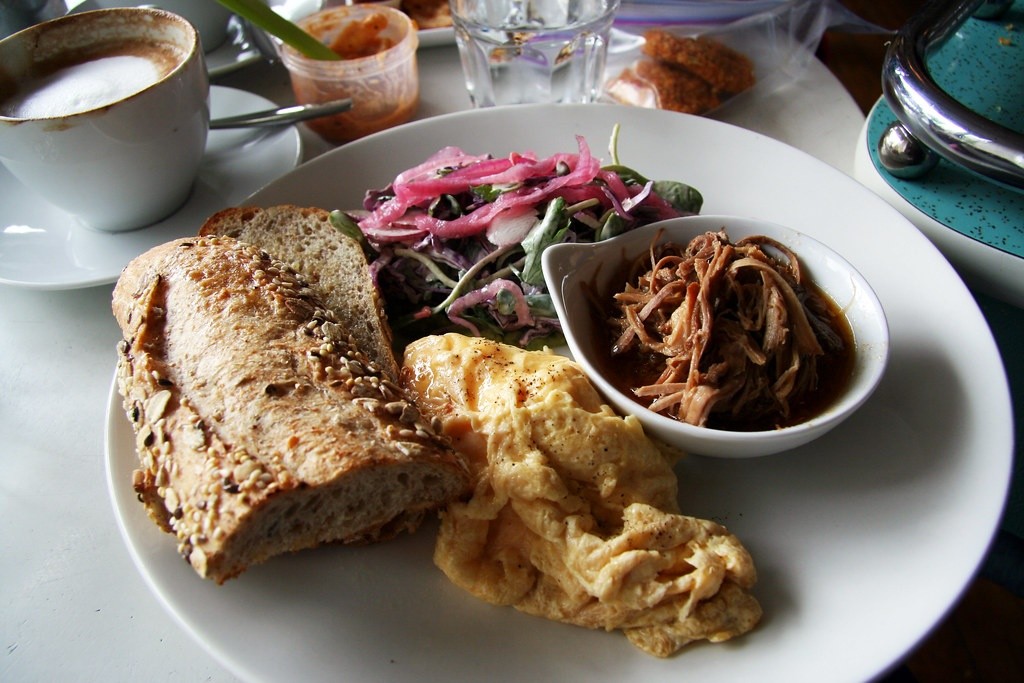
66,0,318,76
103,100,1016,682
0,87,305,292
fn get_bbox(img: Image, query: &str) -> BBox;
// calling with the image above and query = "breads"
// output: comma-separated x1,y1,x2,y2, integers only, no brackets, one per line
111,204,482,580
602,27,755,121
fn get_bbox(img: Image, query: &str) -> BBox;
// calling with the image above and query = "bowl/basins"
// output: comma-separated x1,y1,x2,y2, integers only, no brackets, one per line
540,215,890,459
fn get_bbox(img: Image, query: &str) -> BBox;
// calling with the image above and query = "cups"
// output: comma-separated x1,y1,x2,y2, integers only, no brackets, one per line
282,4,422,143
449,0,622,108
0,6,211,232
96,0,233,53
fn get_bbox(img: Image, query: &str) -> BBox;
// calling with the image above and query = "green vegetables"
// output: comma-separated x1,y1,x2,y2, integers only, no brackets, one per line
326,120,706,350
